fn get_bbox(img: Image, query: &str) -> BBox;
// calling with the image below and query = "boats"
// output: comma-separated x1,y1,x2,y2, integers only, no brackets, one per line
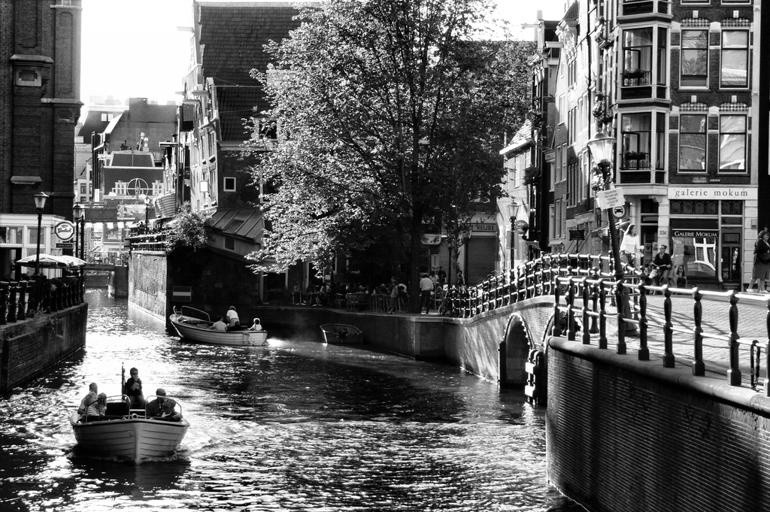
169,314,267,346
70,394,189,466
320,323,363,344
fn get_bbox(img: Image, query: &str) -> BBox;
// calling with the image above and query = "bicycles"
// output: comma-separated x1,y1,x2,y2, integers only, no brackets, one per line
649,263,669,294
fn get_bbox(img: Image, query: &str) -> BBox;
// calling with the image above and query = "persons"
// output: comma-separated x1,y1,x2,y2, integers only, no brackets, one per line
649,244,672,295
620,224,645,268
120,139,129,150
746,229,770,293
208,304,263,332
360,263,465,314
124,367,145,415
78,382,109,422
146,387,182,422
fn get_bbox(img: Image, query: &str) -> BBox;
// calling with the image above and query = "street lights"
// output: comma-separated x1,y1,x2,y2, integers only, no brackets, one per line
506,200,518,278
72,203,82,257
33,192,49,274
462,225,472,283
587,132,637,330
